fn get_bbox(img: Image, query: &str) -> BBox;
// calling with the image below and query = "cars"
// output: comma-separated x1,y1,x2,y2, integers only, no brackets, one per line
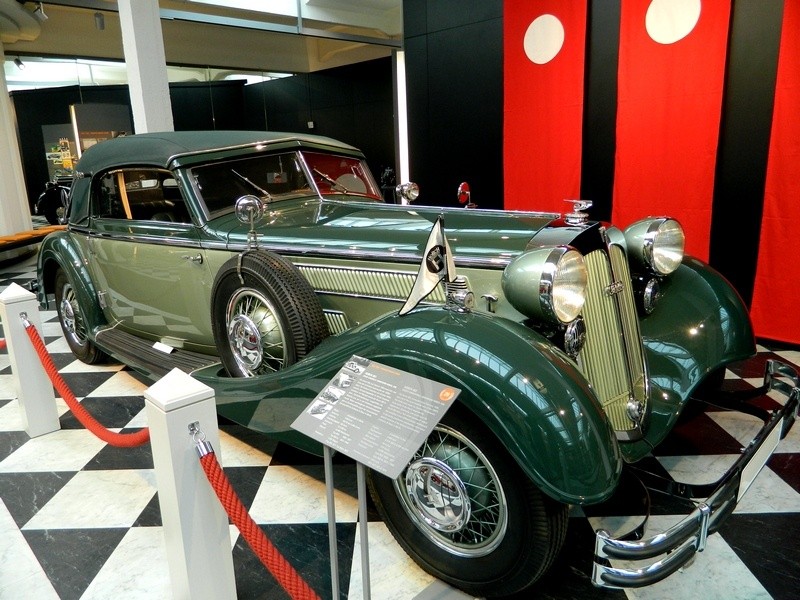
25,130,800,600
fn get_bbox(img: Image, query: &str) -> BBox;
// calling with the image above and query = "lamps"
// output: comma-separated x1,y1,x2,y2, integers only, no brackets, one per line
14,58,25,70
33,2,48,20
94,13,105,31
